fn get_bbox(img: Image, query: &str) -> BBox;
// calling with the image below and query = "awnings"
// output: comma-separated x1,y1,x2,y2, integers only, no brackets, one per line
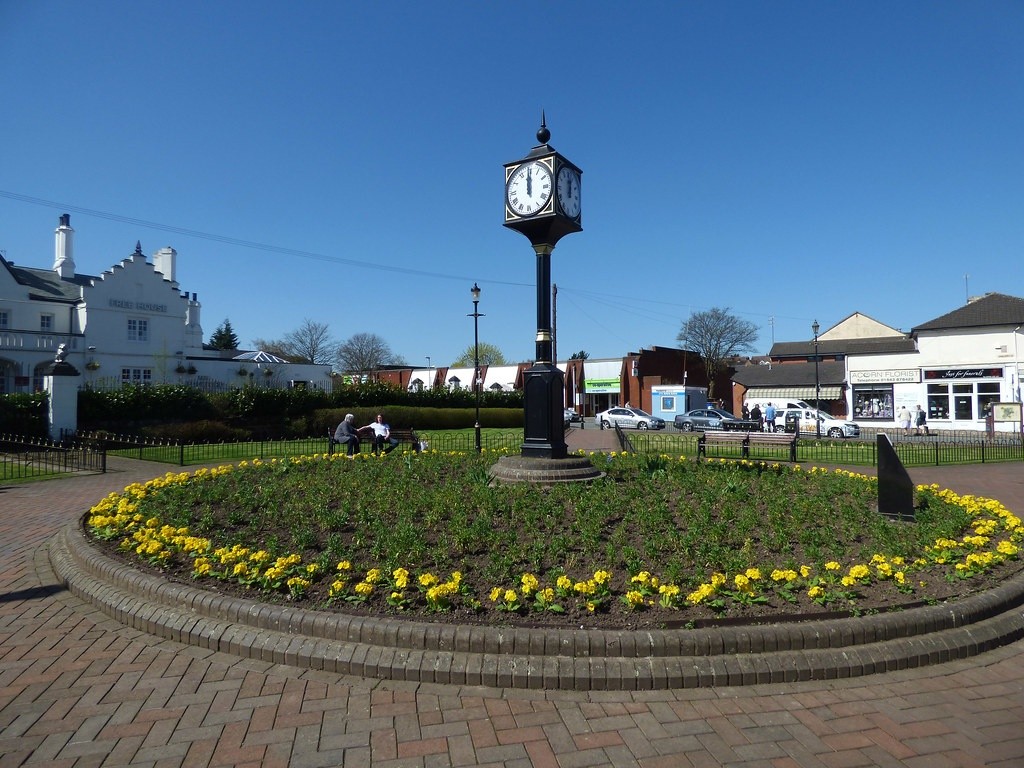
746,387,843,400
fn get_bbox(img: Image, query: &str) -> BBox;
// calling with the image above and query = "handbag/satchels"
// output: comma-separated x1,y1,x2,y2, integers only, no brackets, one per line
411,438,421,453
920,412,926,420
420,441,430,451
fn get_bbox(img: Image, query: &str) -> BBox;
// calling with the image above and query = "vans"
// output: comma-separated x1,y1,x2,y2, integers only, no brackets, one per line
743,398,813,421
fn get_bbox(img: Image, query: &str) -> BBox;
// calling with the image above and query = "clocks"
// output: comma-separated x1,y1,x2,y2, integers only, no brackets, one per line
557,165,581,219
505,160,553,216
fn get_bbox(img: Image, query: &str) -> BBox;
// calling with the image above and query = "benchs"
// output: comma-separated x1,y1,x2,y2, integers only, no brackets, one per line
327,428,418,458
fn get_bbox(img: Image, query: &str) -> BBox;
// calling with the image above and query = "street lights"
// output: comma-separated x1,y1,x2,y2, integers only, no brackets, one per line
809,320,824,438
466,283,486,452
426,357,430,391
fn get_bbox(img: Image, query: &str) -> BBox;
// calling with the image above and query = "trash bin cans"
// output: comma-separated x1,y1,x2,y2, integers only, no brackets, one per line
785,416,799,437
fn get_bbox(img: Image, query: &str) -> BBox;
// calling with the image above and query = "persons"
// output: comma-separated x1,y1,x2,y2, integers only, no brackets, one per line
357,414,399,454
765,402,777,433
334,414,360,458
625,402,630,407
720,398,725,410
741,403,749,419
751,404,764,428
898,406,911,436
914,405,926,435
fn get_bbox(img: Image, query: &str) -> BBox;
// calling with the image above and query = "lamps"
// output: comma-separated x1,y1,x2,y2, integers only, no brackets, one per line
88,346,96,351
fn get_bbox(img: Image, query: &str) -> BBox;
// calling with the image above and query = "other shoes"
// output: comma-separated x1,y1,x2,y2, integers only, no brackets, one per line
347,455,353,458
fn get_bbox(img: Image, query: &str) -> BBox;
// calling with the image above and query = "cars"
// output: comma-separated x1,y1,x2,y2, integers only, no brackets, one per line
763,408,860,438
674,409,738,432
595,407,665,430
564,408,579,422
